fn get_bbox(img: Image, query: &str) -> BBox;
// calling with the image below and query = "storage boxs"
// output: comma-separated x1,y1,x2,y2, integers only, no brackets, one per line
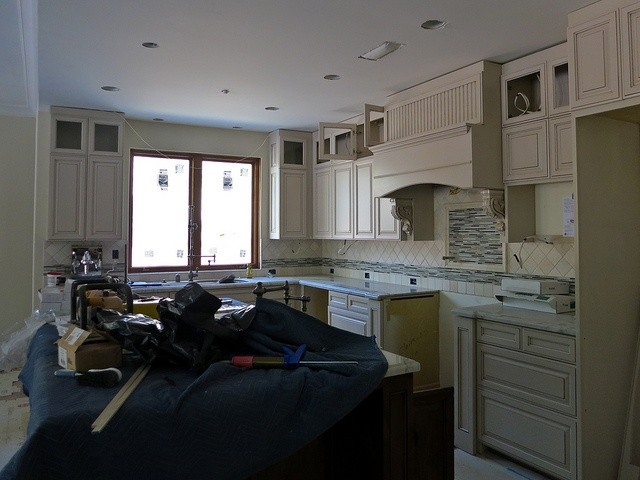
52,322,122,371
132,299,175,319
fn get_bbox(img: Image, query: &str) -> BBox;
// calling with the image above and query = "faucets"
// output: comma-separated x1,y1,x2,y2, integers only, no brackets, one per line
175,274,180,283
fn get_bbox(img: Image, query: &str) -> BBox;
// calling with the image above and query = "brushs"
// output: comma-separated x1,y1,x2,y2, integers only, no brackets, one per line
54,367,123,387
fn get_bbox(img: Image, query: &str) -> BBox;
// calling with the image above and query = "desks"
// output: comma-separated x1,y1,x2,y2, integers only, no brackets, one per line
0,324,420,480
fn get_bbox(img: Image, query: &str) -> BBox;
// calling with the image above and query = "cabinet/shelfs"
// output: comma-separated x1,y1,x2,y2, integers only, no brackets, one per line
452,309,477,453
47,105,125,241
310,116,354,240
499,43,640,182
327,291,440,394
476,319,640,480
320,101,403,241
567,0,640,111
267,130,309,240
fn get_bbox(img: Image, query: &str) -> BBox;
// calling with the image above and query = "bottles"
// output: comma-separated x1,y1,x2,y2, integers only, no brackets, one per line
247,265,252,278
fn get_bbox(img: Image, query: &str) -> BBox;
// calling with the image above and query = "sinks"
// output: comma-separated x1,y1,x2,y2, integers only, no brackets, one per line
198,280,249,283
162,282,188,286
